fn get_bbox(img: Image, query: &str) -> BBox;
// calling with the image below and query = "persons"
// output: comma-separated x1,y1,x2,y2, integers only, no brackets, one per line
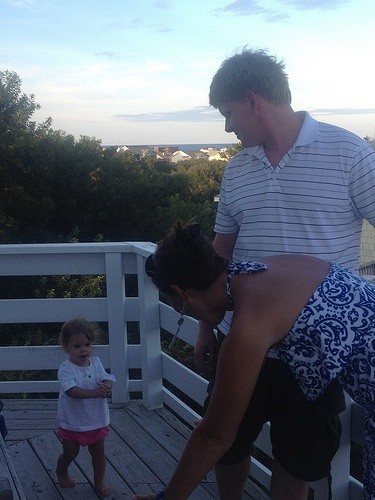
194,51,375,500
145,228,375,500
56,319,117,497
